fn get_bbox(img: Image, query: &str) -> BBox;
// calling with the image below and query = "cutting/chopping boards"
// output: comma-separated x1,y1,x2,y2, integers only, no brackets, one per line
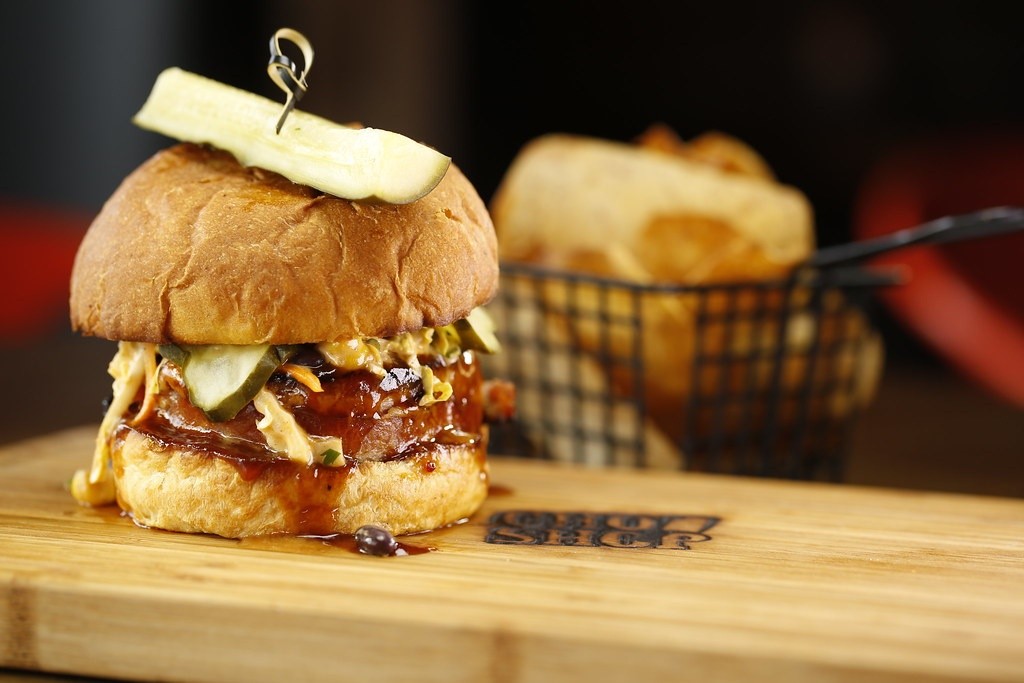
0,426,1023,681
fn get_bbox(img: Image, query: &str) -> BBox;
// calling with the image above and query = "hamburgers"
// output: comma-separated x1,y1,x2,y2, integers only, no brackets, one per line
70,124,500,537
